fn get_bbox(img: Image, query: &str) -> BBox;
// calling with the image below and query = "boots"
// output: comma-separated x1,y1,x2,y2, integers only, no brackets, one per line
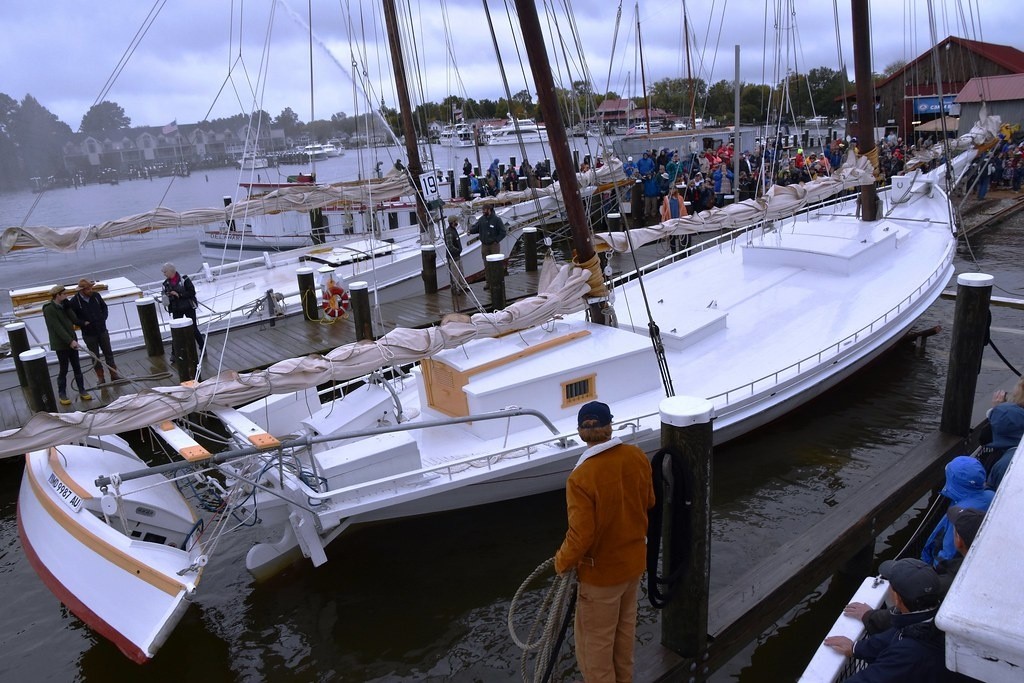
95,366,105,385
108,365,123,381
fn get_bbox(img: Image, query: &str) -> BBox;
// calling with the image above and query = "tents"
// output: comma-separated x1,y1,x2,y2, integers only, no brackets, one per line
914,116,959,131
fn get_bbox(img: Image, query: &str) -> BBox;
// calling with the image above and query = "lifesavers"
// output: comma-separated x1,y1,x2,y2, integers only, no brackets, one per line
320,286,350,319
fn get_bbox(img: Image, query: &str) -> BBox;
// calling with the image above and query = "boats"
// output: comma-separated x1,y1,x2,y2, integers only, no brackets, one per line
795,375,1024,682
197,0,475,262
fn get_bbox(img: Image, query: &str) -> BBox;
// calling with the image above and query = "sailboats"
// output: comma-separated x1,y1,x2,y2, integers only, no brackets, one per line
0,0,527,425
19,0,960,667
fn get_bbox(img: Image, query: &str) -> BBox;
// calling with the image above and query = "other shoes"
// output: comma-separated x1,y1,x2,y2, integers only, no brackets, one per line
59,397,71,404
170,355,176,363
200,350,207,356
80,393,92,400
484,282,490,290
453,290,461,296
459,287,472,292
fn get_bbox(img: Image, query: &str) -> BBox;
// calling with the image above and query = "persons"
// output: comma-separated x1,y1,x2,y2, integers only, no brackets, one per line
463,157,559,198
161,263,207,360
395,159,406,171
70,278,123,385
553,401,656,682
468,203,507,290
43,285,92,404
825,371,1024,683
445,215,472,295
626,131,1023,254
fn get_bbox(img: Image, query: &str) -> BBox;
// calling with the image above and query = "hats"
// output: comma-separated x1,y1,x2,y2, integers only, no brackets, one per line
51,285,69,293
448,215,461,223
948,507,986,546
628,156,633,162
482,204,491,209
820,153,824,156
661,173,669,179
469,173,474,176
798,149,803,153
578,401,614,428
75,279,95,290
878,557,942,603
